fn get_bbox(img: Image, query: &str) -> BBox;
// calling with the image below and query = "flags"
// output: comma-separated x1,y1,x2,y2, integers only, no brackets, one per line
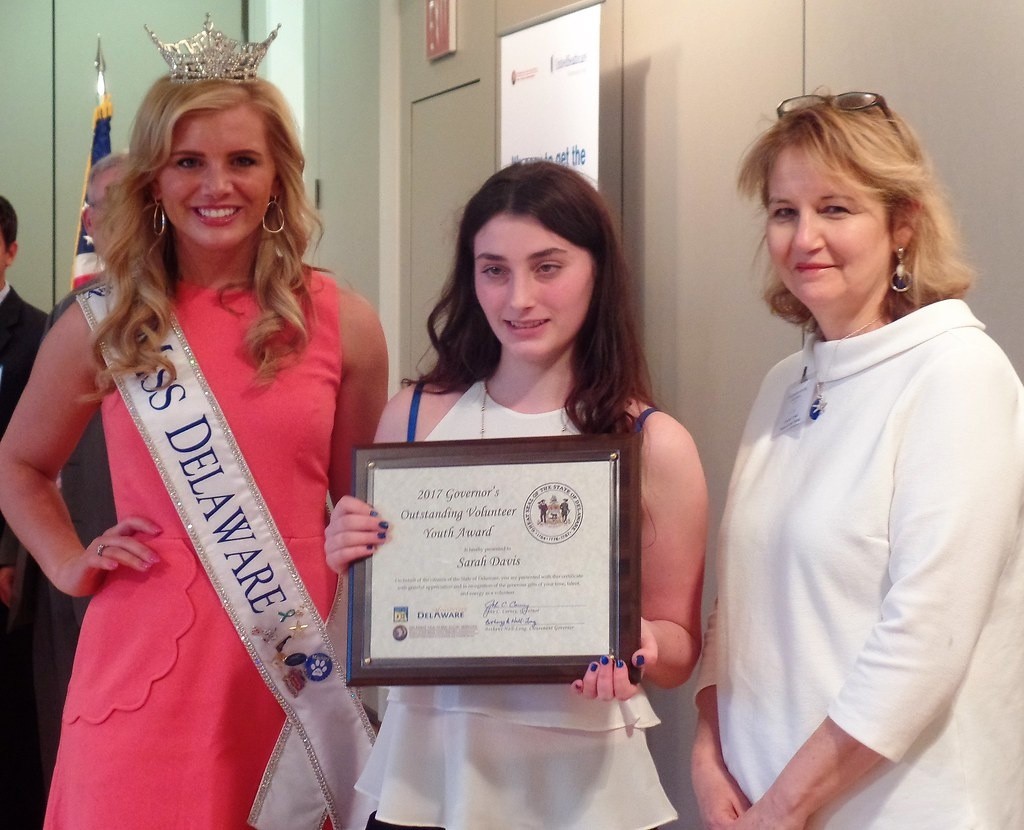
69,104,118,290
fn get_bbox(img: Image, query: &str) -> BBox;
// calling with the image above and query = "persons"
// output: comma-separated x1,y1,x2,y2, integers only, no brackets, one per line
690,88,1024,830
0,196,51,676
21,150,164,809
0,73,390,829
328,157,709,828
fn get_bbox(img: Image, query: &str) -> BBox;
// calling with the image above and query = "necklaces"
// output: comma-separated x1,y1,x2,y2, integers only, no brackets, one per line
801,314,882,416
481,378,572,437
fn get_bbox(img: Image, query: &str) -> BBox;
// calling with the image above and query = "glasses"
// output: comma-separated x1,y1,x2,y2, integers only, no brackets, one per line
777,92,900,133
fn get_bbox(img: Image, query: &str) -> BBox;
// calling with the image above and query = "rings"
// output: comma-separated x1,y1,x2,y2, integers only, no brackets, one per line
97,544,108,560
